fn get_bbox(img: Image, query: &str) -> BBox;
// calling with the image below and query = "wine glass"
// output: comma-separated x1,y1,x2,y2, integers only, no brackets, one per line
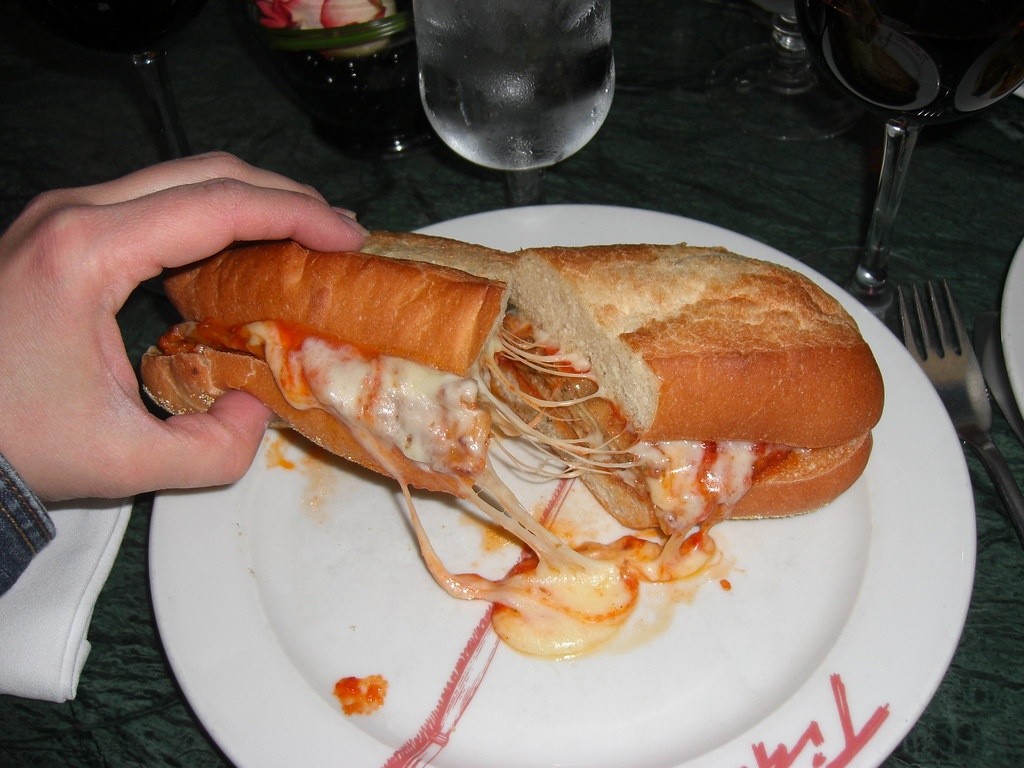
792,0,1023,345
408,1,617,208
42,0,237,162
703,1,867,143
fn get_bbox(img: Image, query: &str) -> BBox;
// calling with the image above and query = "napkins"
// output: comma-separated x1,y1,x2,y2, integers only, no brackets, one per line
1,493,138,704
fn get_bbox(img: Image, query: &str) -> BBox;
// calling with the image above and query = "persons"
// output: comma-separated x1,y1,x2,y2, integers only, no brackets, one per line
0,152,369,597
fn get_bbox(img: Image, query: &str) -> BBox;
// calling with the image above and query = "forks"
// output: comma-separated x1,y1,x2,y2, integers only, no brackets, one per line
896,275,1024,552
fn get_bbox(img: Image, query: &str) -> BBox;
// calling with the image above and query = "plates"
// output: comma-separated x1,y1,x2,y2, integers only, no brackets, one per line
150,203,977,767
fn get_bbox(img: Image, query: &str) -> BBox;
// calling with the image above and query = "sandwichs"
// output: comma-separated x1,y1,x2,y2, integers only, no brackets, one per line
507,239,885,541
140,229,516,502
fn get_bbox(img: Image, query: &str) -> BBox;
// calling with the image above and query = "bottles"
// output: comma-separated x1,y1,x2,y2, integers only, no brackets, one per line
249,0,439,156
